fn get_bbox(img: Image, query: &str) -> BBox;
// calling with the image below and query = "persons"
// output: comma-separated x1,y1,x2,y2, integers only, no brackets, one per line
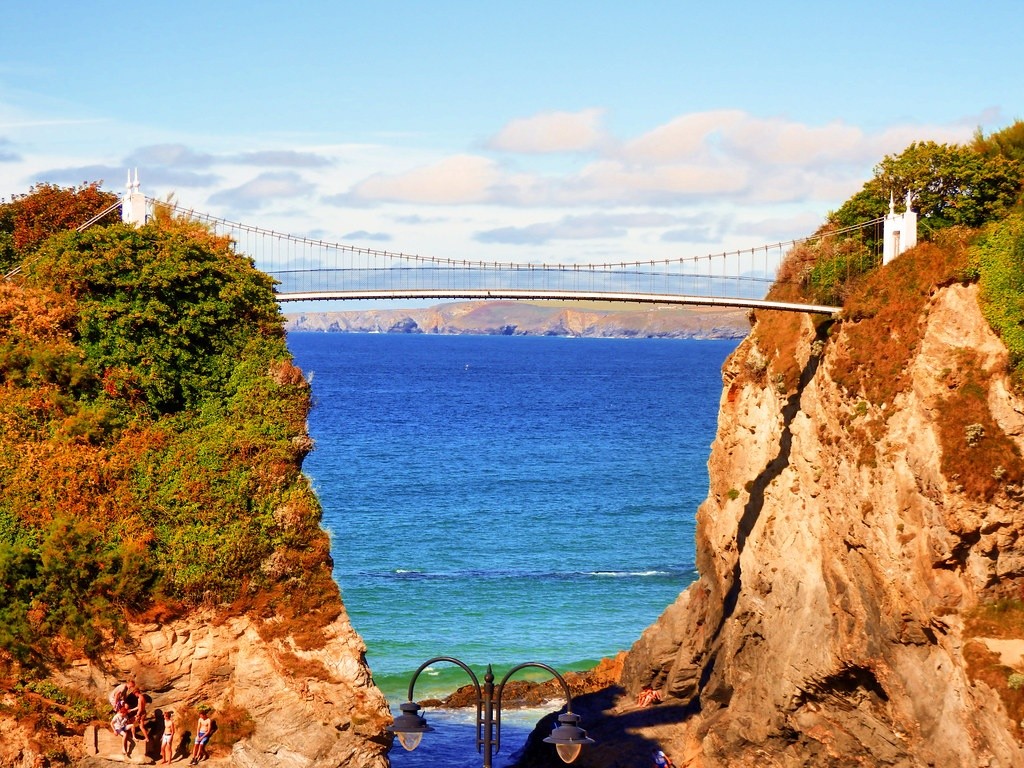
189,707,211,765
652,748,671,768
161,711,174,764
636,687,662,708
109,680,149,754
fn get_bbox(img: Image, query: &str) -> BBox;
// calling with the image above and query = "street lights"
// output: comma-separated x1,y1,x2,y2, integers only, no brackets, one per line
386,657,595,768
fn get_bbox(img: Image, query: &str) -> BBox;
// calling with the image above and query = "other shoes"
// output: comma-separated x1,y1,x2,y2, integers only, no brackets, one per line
190,758,198,765
